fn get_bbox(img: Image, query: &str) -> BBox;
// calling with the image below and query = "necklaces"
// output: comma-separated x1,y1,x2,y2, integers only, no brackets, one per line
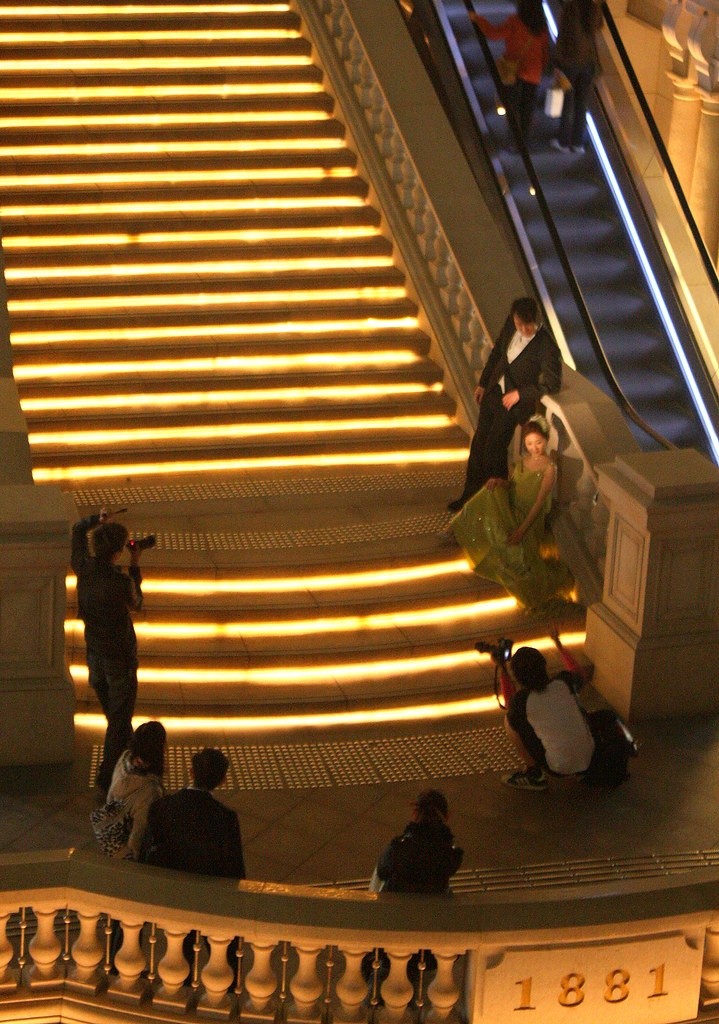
530,454,546,461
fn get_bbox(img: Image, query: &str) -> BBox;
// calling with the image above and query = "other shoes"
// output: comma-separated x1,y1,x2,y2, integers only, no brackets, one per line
506,145,520,155
96,776,112,790
447,500,466,511
550,137,569,151
572,140,586,154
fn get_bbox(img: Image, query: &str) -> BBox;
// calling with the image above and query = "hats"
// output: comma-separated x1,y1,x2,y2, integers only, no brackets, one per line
511,647,550,691
416,788,448,812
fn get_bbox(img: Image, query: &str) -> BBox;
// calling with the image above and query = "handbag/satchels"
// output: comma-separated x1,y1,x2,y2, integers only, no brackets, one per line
579,710,635,788
368,866,385,893
90,780,162,859
496,55,517,85
543,86,565,118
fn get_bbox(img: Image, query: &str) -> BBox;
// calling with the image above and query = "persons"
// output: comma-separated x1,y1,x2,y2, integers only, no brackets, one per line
437,415,567,616
467,0,553,152
139,747,245,995
361,788,466,1007
550,0,607,153
445,296,561,512
70,502,144,792
108,721,168,975
490,621,597,791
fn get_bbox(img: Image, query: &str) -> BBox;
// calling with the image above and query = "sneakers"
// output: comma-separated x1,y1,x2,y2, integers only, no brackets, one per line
499,771,550,791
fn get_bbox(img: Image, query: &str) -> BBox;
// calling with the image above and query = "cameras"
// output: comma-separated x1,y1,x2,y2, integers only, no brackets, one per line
475,638,514,663
127,535,156,553
